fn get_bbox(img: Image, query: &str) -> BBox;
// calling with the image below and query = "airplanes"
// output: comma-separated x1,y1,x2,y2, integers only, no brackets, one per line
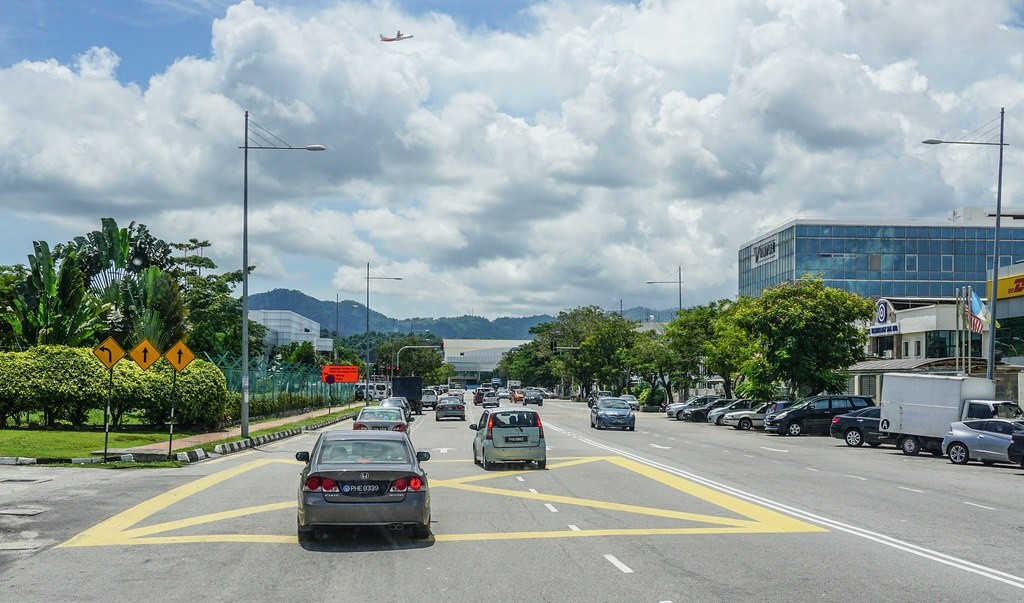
380,30,414,41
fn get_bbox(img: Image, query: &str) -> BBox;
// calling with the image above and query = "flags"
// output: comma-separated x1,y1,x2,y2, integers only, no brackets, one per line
958,291,1001,334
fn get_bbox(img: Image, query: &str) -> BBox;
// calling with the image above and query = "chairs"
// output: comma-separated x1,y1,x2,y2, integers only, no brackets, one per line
330,447,347,460
380,446,394,459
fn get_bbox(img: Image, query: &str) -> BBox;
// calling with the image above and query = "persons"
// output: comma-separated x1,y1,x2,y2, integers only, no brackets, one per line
509,414,529,425
587,391,595,402
509,390,517,403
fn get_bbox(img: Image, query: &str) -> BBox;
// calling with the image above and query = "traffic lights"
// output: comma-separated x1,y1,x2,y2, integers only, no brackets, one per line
440,341,444,350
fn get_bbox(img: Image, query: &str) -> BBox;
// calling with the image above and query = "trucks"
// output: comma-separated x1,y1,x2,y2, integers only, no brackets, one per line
878,373,1024,461
391,375,423,415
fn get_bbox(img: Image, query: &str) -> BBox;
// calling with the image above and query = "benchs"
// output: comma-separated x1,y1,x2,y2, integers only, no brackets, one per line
332,451,397,459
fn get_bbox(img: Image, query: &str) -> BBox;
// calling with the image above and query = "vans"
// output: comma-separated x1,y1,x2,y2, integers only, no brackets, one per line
469,406,546,470
353,382,387,402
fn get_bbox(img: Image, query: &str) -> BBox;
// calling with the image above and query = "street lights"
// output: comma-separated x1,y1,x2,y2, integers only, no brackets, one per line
333,293,359,365
238,110,327,435
365,261,403,405
645,266,684,318
921,106,1010,380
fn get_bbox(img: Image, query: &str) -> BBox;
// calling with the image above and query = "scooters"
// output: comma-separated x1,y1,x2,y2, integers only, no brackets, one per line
587,397,596,409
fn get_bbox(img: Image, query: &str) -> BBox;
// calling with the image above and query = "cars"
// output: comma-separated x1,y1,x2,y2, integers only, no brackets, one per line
830,406,907,450
665,394,795,430
590,397,636,431
764,394,876,437
618,395,640,411
295,428,430,543
1007,429,1024,467
941,418,1024,468
421,377,556,408
596,391,612,400
378,396,412,421
435,396,467,421
352,406,415,439
